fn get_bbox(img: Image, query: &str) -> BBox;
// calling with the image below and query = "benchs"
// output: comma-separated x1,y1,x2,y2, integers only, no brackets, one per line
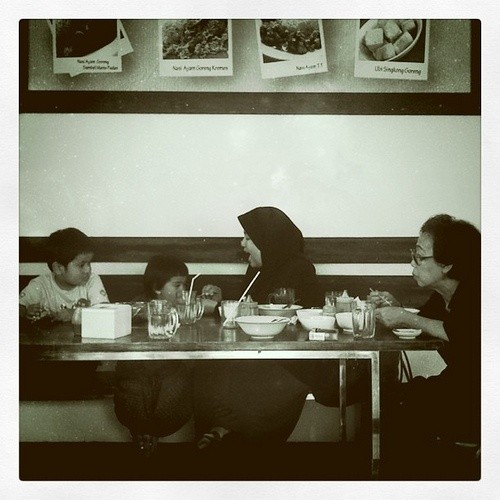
19,236,435,441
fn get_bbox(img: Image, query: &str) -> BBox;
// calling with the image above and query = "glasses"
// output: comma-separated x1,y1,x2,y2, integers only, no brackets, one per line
407,247,433,265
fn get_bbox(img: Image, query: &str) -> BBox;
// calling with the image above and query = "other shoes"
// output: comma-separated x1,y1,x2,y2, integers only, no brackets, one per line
130,431,158,457
192,429,233,453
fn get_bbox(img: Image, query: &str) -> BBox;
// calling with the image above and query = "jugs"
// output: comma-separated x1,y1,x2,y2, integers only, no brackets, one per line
71,298,91,336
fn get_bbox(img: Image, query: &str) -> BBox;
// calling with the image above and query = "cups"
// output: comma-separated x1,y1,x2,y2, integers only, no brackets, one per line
352,306,375,338
267,288,295,304
177,298,205,324
324,290,342,314
239,302,258,316
147,300,178,339
221,300,238,329
182,291,197,324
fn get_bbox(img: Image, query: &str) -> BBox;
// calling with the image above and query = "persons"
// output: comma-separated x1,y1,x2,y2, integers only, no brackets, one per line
190,206,326,481
19,227,110,481
360,215,481,481
115,253,196,458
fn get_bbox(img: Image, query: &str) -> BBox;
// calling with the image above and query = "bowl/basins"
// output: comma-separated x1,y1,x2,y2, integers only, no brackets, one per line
296,309,335,330
235,316,290,338
335,312,353,332
258,304,303,318
392,328,422,339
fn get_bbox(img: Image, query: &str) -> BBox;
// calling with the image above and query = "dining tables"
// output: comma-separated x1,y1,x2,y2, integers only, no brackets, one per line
19,314,444,481
